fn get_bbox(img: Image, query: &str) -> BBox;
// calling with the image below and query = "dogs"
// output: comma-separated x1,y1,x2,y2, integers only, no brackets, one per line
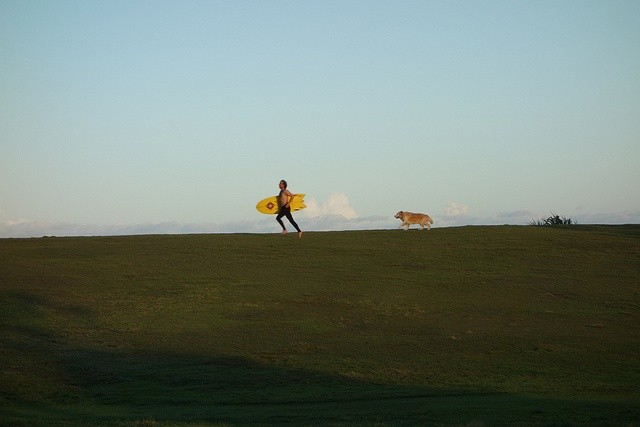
394,210,434,231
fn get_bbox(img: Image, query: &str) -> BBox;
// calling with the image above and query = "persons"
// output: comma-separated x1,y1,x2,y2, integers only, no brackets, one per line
276,180,303,239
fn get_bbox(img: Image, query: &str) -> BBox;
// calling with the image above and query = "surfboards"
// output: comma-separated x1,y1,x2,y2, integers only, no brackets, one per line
256,194,307,214
